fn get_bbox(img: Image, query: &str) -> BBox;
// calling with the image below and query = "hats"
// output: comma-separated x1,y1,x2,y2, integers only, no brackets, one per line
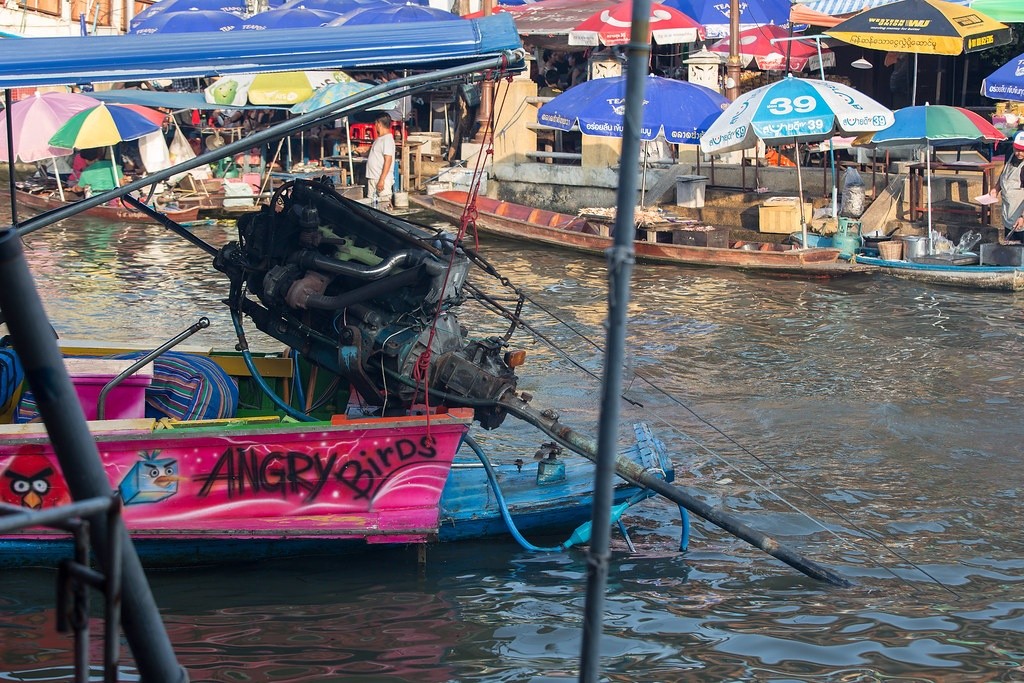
1012,131,1024,151
205,135,226,152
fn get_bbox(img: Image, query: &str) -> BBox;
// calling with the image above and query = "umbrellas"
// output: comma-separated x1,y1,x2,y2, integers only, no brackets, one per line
822,0,1013,106
0,90,101,201
538,73,734,211
707,21,836,85
699,73,895,248
661,0,809,88
805,0,1024,108
568,0,705,46
127,0,464,34
980,53,1024,103
289,81,396,185
204,71,358,170
851,102,1009,256
48,101,160,188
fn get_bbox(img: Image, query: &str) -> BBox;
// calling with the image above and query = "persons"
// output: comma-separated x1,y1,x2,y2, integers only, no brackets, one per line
989,132,1024,244
63,147,126,199
110,76,225,126
523,41,588,162
209,109,348,173
353,69,411,136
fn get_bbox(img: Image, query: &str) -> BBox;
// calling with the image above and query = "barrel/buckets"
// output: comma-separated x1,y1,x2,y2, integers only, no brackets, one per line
901,235,930,261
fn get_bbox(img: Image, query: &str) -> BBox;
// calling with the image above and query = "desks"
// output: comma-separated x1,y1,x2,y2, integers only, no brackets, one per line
908,161,992,227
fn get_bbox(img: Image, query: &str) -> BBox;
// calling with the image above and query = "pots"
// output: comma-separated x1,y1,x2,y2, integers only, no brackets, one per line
862,226,900,242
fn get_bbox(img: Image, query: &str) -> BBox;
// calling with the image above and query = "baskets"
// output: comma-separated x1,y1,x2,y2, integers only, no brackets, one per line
876,241,903,261
888,221,924,242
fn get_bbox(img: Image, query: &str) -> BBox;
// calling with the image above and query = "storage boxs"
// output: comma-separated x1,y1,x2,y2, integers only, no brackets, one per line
756,203,812,234
672,226,730,249
408,136,488,196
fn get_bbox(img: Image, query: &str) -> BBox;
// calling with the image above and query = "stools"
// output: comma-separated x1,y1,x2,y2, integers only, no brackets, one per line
390,121,408,142
407,108,418,129
64,355,153,420
350,122,377,140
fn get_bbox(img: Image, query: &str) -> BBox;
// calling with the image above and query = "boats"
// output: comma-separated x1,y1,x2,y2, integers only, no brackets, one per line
410,190,882,281
0,171,853,578
789,231,1024,294
8,162,366,227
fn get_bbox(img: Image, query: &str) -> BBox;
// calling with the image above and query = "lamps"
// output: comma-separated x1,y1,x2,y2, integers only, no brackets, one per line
851,49,873,69
